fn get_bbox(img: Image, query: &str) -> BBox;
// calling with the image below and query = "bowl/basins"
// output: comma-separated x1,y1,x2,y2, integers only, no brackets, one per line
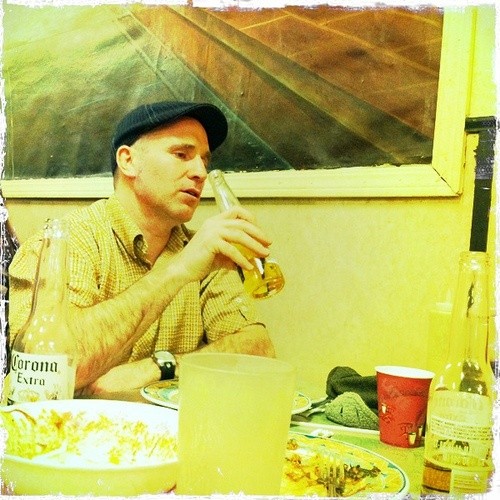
2,398,182,495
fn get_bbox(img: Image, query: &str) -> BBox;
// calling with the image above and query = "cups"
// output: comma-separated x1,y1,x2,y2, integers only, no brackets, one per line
375,365,435,448
178,350,294,494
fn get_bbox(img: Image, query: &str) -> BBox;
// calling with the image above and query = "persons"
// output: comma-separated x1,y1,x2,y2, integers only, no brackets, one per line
9,100,276,398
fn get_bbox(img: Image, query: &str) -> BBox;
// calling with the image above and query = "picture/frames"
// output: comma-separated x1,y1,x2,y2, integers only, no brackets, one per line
0,3,474,200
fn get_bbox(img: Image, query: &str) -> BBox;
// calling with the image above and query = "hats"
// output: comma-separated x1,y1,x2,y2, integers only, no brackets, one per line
111,102,228,174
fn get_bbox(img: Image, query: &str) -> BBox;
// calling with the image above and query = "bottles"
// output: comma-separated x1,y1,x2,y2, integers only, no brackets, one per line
207,169,285,301
419,249,496,500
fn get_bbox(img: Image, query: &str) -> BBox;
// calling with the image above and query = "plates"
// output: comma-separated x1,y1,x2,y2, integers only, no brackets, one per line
285,427,411,499
141,376,313,416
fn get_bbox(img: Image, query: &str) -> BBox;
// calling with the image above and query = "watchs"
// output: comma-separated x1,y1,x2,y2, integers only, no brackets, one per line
153,350,176,381
